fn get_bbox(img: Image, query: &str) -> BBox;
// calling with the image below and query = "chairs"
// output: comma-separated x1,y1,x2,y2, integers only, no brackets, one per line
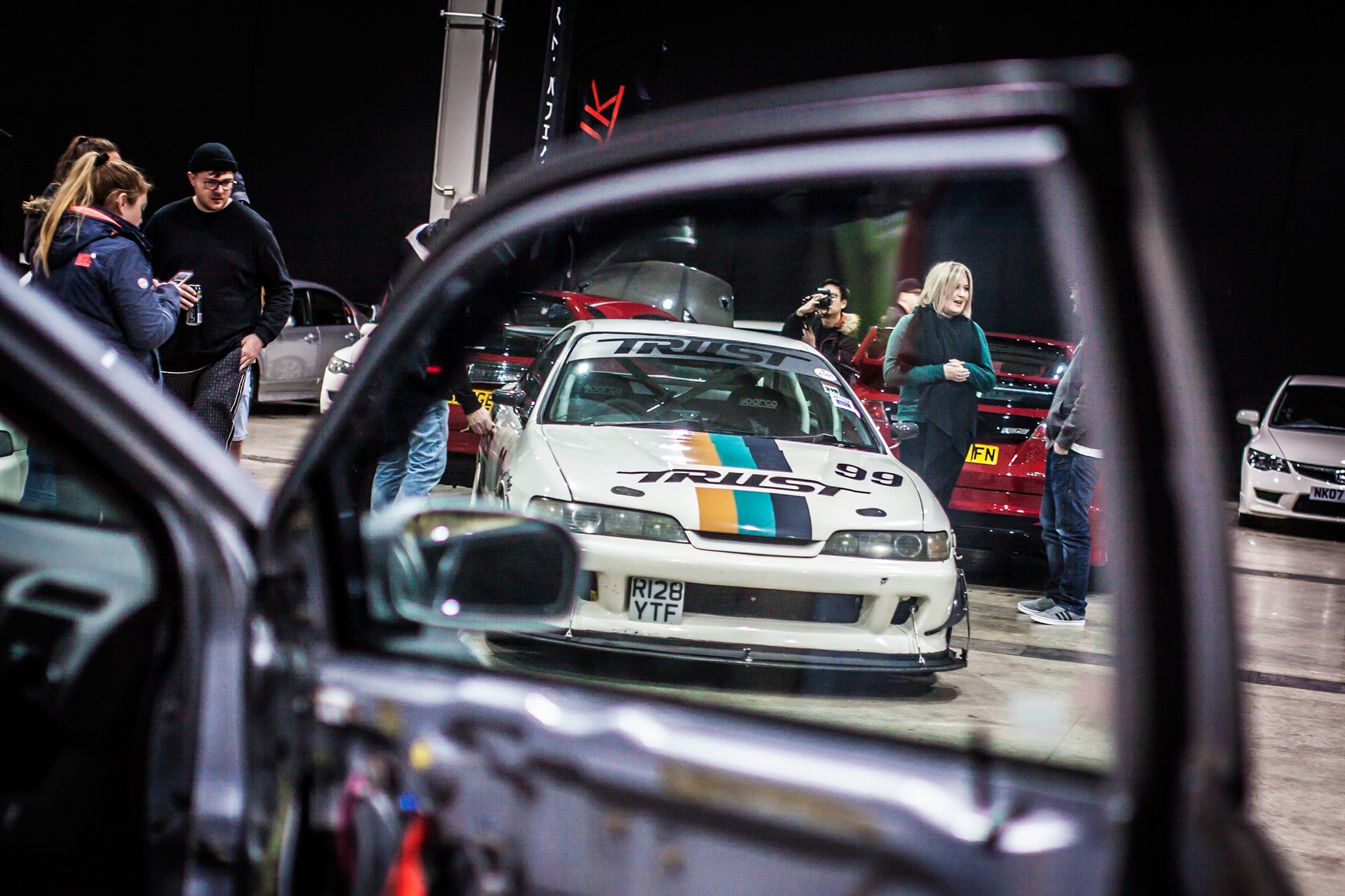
567,372,637,424
716,385,793,439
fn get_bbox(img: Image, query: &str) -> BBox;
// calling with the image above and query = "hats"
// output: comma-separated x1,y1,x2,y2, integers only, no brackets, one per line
229,171,250,205
188,142,237,174
897,278,923,296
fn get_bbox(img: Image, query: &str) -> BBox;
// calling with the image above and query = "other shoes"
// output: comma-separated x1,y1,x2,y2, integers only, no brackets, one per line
1017,597,1057,615
1030,606,1085,626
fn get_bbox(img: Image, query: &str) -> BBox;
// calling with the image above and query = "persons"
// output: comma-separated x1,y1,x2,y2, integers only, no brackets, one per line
1017,278,1107,625
19,133,125,516
881,261,996,514
30,146,183,531
366,194,497,517
141,142,296,456
878,276,924,331
779,278,862,385
224,167,254,467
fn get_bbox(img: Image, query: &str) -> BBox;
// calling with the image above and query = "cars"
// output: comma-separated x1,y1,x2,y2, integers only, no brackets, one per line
1235,373,1345,528
320,322,378,414
248,277,381,411
0,412,28,505
445,260,735,455
470,309,970,677
842,330,1111,593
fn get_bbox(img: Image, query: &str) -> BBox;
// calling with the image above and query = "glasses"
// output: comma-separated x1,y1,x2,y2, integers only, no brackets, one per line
195,175,237,192
907,291,922,295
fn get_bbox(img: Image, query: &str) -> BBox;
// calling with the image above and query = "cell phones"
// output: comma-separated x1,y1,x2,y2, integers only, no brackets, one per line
168,271,193,287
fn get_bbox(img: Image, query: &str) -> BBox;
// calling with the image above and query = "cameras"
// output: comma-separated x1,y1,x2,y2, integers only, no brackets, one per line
815,287,831,309
